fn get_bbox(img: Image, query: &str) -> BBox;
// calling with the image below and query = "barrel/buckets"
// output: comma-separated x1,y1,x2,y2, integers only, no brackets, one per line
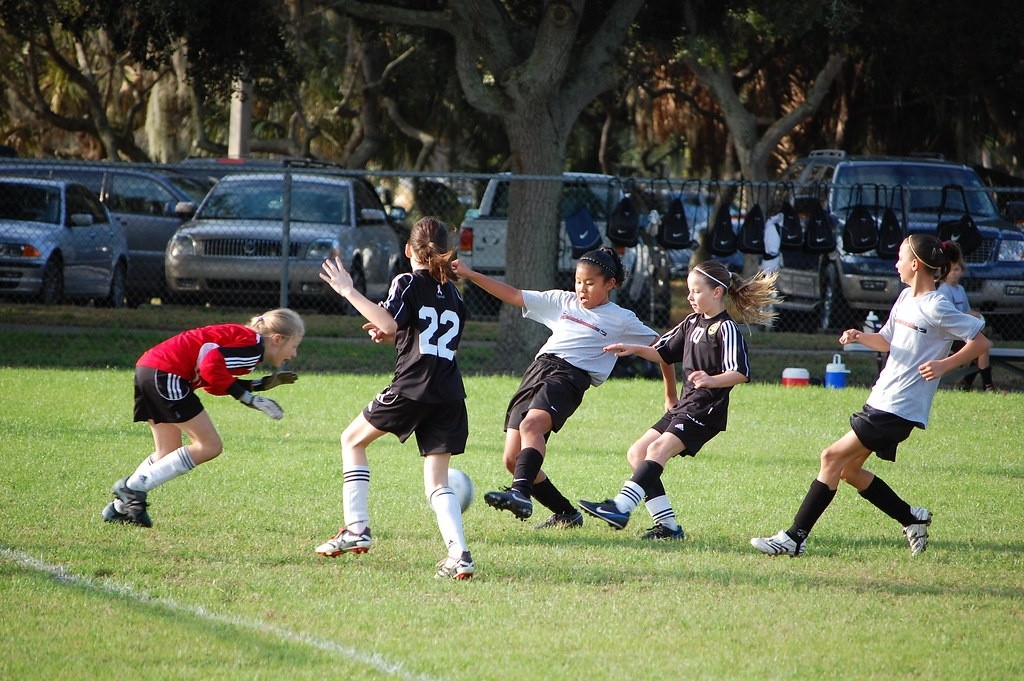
781,368,809,387
825,353,850,390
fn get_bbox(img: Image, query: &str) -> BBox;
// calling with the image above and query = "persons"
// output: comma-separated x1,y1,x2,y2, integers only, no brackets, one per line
578,261,786,537
750,233,994,558
450,247,679,529
102,306,304,527
315,215,473,581
937,256,1009,395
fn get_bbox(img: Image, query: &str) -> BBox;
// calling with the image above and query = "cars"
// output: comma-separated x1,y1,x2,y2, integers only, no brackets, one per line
0,175,133,310
165,166,408,318
639,188,745,242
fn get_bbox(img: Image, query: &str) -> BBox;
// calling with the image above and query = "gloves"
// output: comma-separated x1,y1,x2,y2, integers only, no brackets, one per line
250,370,298,392
240,390,284,420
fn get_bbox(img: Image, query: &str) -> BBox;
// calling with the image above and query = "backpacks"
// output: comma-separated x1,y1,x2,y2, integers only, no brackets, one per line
566,175,982,272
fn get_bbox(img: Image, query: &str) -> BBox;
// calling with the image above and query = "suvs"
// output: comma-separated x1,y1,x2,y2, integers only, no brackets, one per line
0,158,280,294
764,148,1024,331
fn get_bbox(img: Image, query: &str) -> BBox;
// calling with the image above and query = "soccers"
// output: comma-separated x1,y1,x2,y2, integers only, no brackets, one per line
431,469,472,514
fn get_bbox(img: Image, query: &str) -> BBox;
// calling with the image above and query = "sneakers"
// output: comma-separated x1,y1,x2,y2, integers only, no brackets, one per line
434,551,475,581
536,510,584,529
102,501,138,524
750,529,807,556
484,486,533,521
111,476,152,528
316,527,372,558
577,499,630,530
641,522,684,541
903,506,933,558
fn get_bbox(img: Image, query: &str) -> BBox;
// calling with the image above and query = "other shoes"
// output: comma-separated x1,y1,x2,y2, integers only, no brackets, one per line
984,385,1011,395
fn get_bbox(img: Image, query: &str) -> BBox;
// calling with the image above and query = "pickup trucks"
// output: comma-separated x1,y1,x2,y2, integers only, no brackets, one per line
457,173,626,314
